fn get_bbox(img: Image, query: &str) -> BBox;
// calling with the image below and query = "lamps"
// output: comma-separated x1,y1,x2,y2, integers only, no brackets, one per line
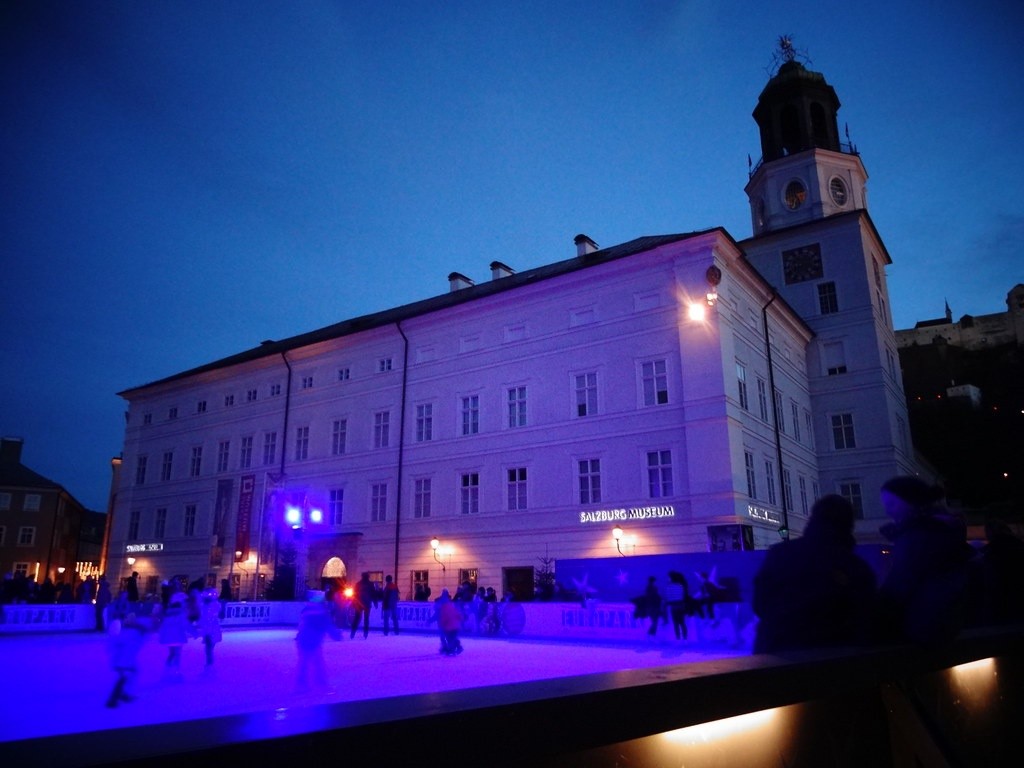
430,536,445,571
778,525,791,539
613,525,627,557
127,556,135,571
235,550,248,577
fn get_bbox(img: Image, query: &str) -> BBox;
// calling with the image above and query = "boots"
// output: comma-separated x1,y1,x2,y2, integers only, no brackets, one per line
103,677,135,709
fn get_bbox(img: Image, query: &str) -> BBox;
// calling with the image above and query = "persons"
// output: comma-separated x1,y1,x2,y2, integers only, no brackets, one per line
634,477,1024,768
0,573,593,706
